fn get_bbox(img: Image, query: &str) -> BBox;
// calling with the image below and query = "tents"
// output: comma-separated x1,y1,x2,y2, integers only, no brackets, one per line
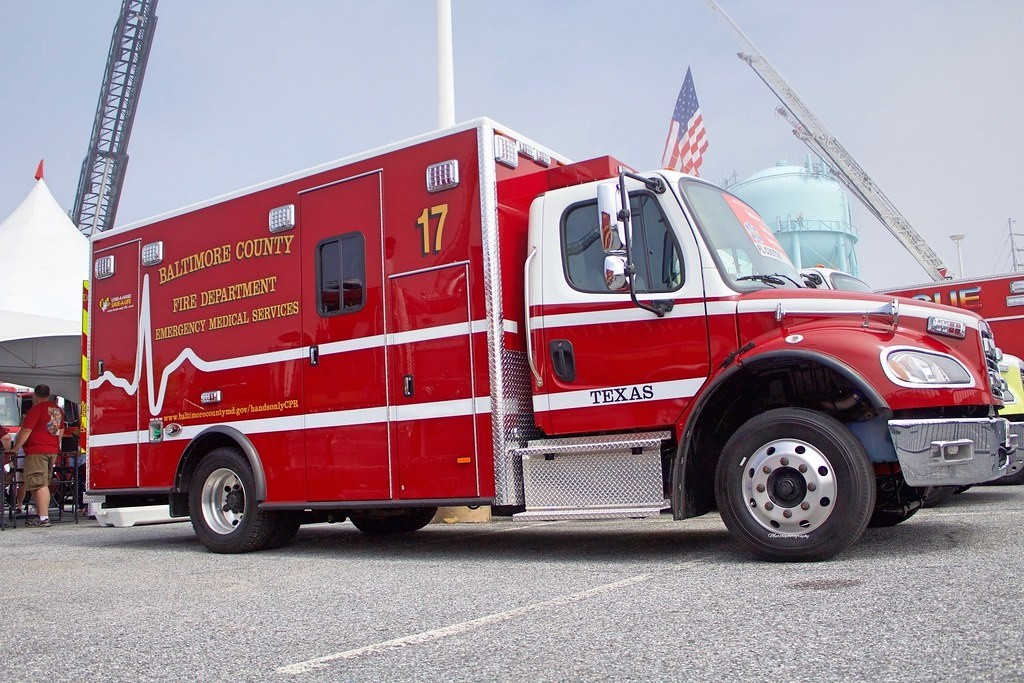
0,160,91,405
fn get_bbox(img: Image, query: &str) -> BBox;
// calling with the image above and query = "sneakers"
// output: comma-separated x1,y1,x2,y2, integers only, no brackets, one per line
24,517,52,528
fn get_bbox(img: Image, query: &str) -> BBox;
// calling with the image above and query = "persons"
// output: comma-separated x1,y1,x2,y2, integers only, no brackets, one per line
8,384,64,528
0,412,26,516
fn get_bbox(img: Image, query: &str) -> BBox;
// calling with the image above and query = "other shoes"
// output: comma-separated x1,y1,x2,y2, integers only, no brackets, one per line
14,509,22,514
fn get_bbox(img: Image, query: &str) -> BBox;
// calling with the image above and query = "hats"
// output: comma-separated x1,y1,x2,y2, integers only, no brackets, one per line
35,384,50,397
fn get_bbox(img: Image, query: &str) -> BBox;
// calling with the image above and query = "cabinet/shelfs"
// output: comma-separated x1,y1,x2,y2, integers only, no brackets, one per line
9,437,78,528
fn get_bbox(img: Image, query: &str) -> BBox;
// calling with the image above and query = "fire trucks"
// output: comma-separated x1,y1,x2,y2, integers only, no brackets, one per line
77,116,1023,564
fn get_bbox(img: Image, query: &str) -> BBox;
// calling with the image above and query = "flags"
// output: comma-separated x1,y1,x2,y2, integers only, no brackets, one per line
660,64,708,178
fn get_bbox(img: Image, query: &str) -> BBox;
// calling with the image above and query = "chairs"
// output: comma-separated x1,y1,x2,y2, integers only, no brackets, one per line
589,266,646,291
